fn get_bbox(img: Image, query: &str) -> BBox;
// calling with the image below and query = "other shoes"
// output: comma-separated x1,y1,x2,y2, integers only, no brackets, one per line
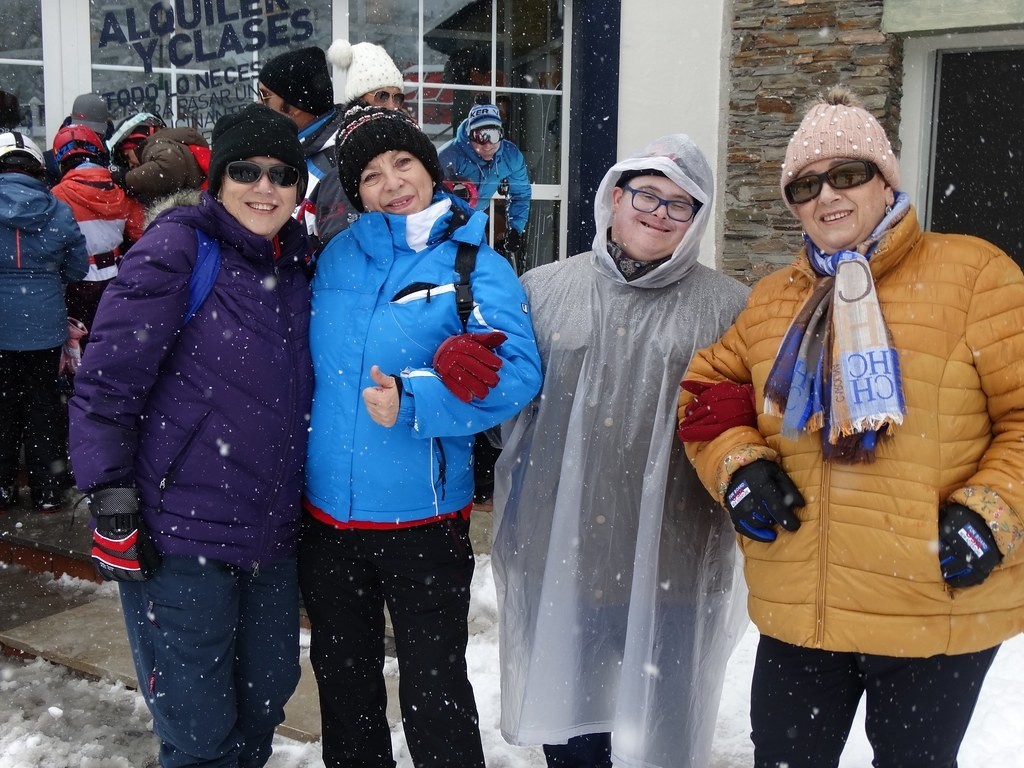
1,484,21,509
31,485,74,513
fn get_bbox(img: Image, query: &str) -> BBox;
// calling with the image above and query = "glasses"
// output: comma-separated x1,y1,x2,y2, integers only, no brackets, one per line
367,92,405,106
260,89,277,104
784,158,878,205
225,161,300,187
623,183,697,223
478,131,500,144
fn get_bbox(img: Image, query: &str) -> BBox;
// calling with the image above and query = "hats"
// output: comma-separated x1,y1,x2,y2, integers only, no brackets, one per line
208,104,308,203
71,92,108,135
259,46,334,114
613,166,703,218
468,107,503,135
333,106,445,211
328,40,404,106
777,90,903,222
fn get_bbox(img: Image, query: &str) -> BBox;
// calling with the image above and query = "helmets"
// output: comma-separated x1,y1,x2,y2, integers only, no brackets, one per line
52,126,106,172
0,130,46,170
106,111,163,167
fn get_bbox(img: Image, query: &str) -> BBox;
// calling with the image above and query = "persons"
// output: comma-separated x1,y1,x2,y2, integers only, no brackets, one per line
0,39,405,511
678,83,1024,768
432,133,750,768
436,104,531,254
301,97,544,768
68,101,316,768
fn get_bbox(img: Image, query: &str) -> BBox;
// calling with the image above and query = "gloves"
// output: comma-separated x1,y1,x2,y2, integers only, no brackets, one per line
725,461,806,544
433,332,508,402
934,501,1002,591
57,314,89,375
114,170,129,188
675,378,760,442
84,487,162,583
503,227,522,255
